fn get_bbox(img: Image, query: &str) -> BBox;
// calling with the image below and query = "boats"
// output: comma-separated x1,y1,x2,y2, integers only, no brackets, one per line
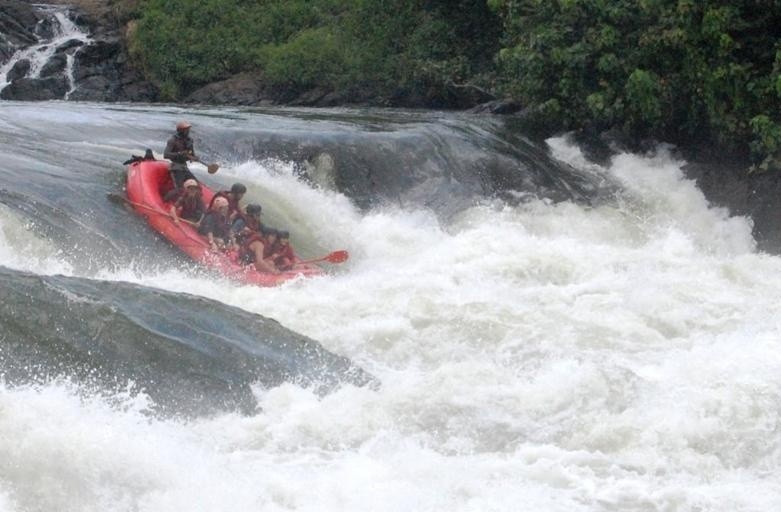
123,149,328,289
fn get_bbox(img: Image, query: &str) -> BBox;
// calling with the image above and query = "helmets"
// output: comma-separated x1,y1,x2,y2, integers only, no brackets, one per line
263,227,290,239
231,183,247,194
183,179,198,189
176,120,192,129
246,203,261,214
212,195,229,210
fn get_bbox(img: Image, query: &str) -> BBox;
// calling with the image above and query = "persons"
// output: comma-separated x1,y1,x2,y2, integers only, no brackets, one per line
162,122,203,202
171,178,298,275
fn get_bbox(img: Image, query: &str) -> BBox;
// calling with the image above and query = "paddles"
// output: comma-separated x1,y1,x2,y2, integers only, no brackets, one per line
295,250,348,265
106,192,197,228
185,152,219,174
